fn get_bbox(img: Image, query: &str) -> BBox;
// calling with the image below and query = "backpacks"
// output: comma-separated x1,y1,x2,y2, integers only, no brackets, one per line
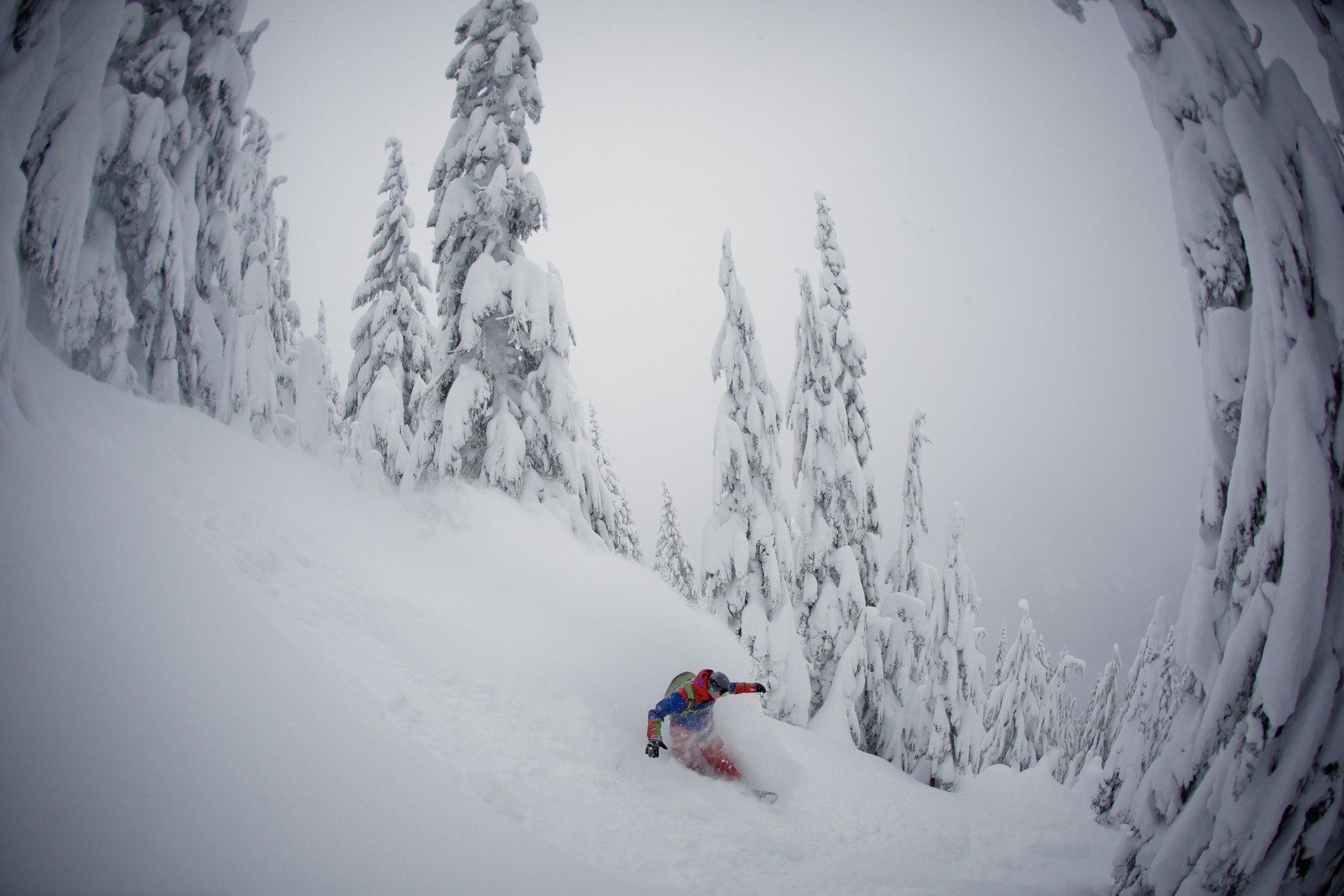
664,672,697,716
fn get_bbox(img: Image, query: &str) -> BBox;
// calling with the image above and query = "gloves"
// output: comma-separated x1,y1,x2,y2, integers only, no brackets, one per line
645,740,667,758
755,683,766,693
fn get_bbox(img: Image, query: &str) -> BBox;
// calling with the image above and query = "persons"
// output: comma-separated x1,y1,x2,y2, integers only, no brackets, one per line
645,669,766,799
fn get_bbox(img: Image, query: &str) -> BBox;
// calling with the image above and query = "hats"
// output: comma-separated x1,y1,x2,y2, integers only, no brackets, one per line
707,671,730,691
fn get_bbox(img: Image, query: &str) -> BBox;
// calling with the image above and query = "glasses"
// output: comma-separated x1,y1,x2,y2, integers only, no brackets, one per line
718,690,730,697
